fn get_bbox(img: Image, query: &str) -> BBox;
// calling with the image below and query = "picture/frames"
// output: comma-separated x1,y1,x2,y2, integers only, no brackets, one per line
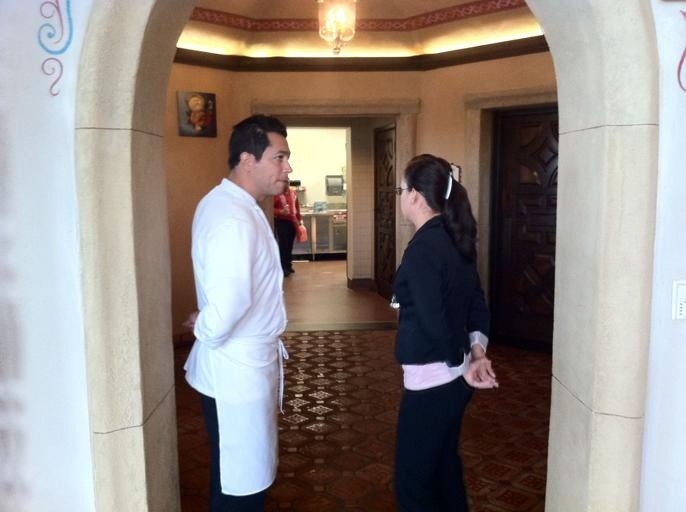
177,91,217,137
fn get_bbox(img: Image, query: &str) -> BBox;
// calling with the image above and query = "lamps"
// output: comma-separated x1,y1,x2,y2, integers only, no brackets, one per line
317,0,357,52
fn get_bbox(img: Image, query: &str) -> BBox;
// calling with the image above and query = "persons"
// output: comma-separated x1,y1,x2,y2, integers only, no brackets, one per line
273,178,305,279
394,153,499,512
182,113,293,511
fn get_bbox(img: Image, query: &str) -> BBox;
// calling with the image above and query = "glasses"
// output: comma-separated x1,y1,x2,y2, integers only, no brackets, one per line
391,184,411,196
